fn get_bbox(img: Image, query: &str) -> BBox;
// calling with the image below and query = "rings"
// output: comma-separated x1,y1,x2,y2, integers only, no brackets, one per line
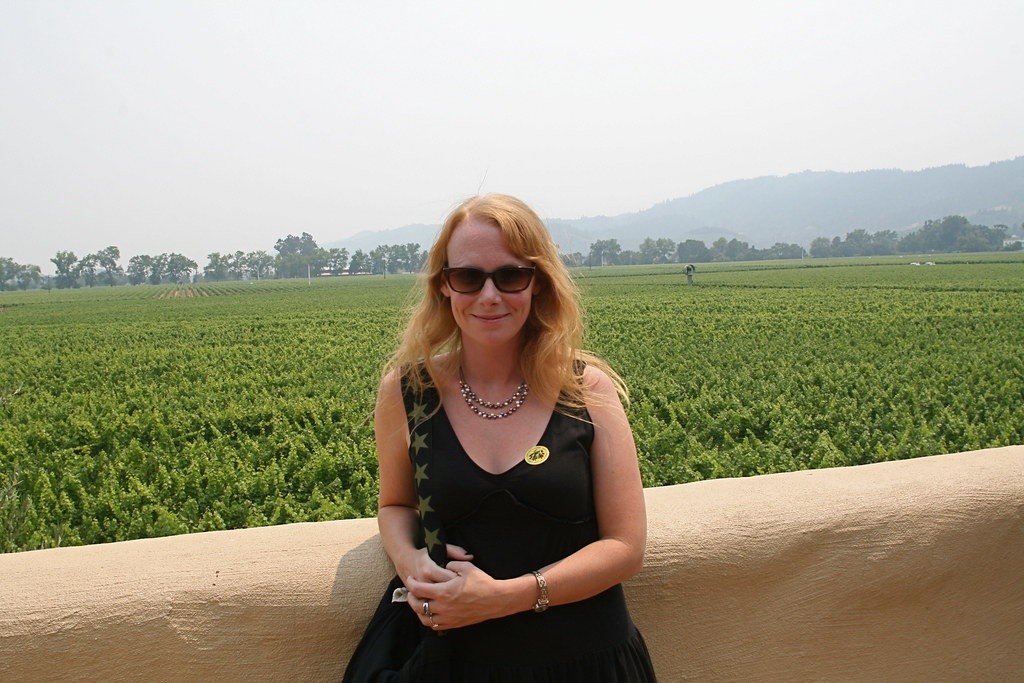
424,600,432,615
430,616,438,628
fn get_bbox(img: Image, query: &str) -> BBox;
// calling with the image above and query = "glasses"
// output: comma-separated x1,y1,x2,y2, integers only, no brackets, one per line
442,262,536,293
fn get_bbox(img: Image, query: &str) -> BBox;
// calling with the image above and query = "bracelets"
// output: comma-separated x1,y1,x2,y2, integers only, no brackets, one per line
531,571,549,611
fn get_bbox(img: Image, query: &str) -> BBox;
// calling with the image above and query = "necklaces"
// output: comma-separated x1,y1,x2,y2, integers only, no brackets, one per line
457,362,528,419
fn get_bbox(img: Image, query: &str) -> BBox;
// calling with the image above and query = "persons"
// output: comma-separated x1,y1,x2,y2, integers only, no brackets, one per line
340,194,655,683
